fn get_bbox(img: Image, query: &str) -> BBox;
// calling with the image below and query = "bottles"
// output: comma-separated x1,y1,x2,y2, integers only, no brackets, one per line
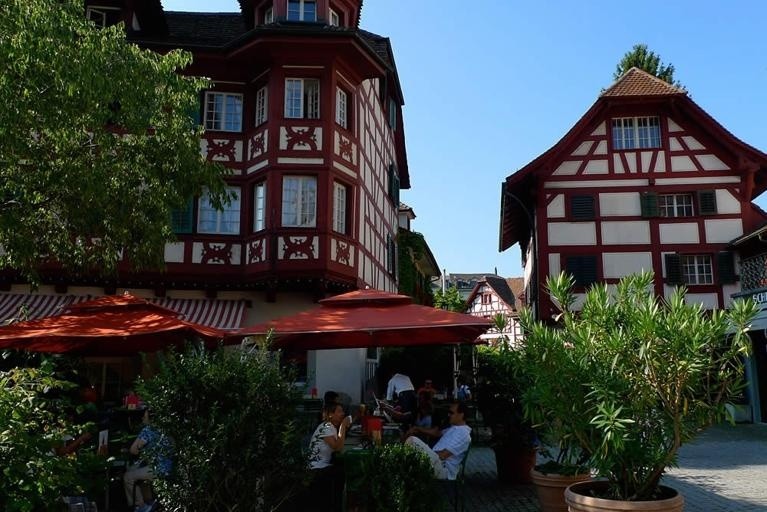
311,384,317,399
432,391,447,401
373,408,379,416
126,390,138,411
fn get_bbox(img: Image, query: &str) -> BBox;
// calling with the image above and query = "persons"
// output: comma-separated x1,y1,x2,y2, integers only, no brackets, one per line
306,369,471,502
44,417,92,480
122,411,172,512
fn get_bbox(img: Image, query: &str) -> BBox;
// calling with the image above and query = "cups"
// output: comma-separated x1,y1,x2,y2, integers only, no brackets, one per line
346,415,352,423
359,404,365,415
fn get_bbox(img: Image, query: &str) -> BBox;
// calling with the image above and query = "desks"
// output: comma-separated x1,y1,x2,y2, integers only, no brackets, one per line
338,433,417,457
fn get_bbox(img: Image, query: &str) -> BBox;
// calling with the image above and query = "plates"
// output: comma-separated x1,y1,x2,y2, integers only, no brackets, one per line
351,447,362,450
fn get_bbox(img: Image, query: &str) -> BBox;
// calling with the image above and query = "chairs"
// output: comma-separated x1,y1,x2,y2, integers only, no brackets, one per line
430,440,475,512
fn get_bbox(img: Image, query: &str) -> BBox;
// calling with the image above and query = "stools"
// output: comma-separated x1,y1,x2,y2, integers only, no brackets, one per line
128,478,160,510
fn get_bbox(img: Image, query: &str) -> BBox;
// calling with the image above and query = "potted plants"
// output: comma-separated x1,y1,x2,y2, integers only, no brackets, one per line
475,303,592,511
474,366,539,487
511,270,762,512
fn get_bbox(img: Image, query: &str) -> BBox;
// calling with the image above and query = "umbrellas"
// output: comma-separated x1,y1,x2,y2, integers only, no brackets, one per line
225,286,490,358
0,290,230,394
